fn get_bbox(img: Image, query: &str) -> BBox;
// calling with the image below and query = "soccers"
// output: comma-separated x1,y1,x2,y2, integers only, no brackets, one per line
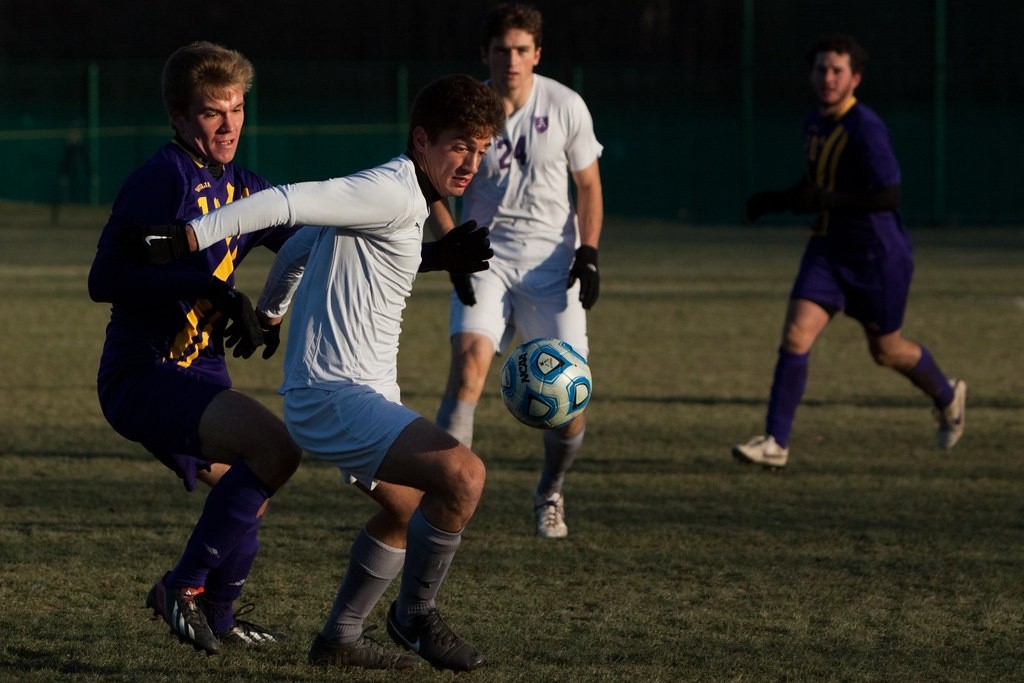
500,337,592,431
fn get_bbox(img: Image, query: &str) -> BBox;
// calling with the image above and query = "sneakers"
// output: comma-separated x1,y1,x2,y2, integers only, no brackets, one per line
386,599,486,670
218,616,294,646
933,377,967,449
307,632,421,670
145,569,222,654
732,433,789,469
535,491,568,538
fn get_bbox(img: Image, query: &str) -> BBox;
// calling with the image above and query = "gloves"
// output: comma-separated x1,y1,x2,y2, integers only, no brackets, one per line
449,273,477,306
417,220,494,274
566,244,600,311
782,181,830,216
108,220,192,271
739,188,790,231
205,276,264,360
253,307,283,360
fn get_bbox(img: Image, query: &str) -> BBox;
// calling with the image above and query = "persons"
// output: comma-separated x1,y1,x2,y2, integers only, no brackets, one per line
731,40,968,467
112,73,505,674
432,7,606,540
88,43,495,654
